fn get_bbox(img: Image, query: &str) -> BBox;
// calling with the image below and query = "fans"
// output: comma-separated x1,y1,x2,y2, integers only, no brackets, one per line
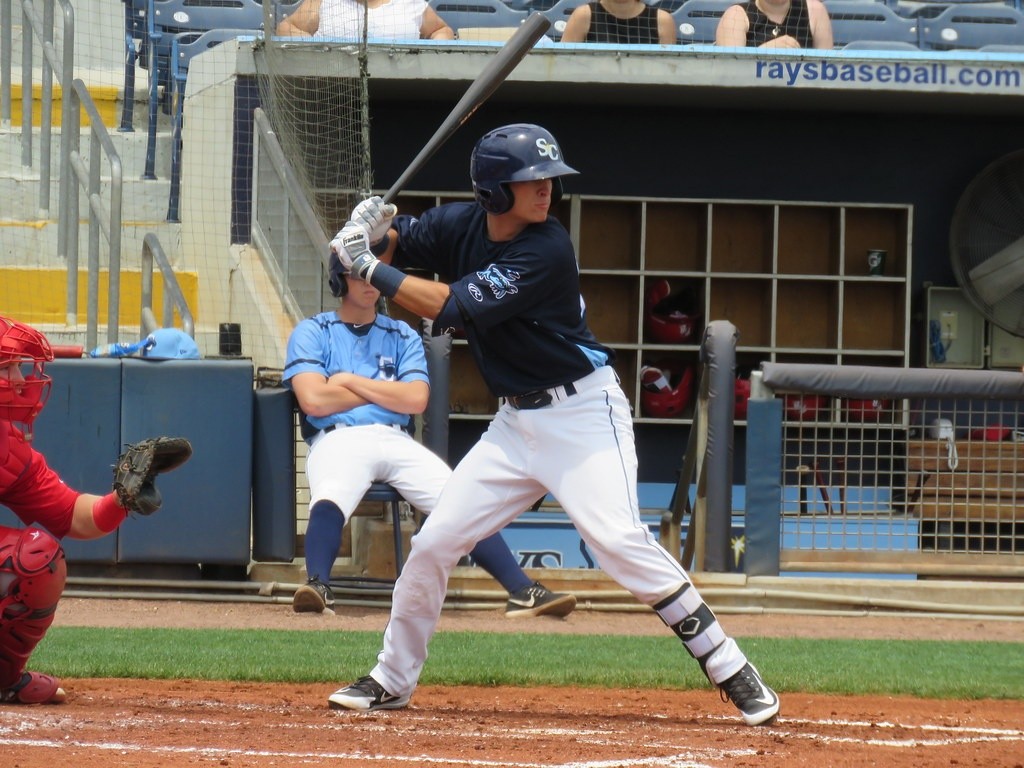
947,147,1024,340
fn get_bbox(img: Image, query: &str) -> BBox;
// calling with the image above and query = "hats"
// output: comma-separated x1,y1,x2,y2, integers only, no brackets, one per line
137,328,200,360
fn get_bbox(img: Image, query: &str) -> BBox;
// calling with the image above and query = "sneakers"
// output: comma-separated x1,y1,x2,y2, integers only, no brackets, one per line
328,675,411,712
504,579,576,619
718,661,780,726
294,573,336,615
0,671,58,704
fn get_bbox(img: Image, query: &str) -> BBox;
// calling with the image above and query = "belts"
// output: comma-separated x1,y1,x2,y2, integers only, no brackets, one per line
324,424,408,434
502,382,577,410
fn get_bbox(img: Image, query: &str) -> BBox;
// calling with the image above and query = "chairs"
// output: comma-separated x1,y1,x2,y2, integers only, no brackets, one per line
299,409,417,590
116,0,1024,224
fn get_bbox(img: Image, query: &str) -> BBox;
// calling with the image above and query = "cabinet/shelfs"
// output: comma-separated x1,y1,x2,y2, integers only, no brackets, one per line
308,186,915,430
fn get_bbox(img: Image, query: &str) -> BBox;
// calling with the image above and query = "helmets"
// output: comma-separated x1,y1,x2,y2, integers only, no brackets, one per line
644,283,701,345
733,365,752,421
470,123,581,214
639,361,693,418
328,251,356,297
840,397,892,423
777,391,829,421
0,312,54,424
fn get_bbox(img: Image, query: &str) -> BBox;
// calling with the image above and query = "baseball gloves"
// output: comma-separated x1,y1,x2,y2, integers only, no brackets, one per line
110,435,194,517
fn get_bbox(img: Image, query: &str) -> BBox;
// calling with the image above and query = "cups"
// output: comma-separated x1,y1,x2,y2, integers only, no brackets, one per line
219,323,242,356
865,248,888,277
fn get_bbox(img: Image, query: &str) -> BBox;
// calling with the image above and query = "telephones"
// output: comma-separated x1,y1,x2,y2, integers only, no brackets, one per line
931,419,953,440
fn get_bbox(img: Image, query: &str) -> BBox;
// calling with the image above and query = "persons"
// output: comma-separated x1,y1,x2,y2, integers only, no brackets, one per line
560,0,677,43
716,0,834,49
282,251,578,616
329,124,782,723
0,317,193,706
276,0,452,40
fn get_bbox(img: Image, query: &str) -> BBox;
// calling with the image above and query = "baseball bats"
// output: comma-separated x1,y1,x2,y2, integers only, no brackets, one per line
812,461,834,514
382,8,552,201
907,471,930,513
837,460,846,514
796,465,809,514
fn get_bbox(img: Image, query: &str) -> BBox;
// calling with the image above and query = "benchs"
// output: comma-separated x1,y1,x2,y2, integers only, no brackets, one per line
905,439,1024,550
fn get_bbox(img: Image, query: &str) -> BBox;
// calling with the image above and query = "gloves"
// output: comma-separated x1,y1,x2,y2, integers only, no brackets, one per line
329,221,408,299
350,197,399,256
